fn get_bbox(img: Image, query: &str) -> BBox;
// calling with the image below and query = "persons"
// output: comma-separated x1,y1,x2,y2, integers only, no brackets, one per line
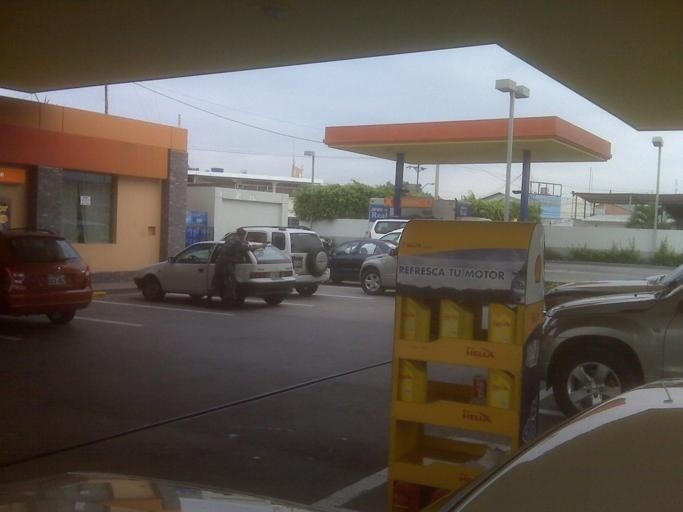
213,228,268,309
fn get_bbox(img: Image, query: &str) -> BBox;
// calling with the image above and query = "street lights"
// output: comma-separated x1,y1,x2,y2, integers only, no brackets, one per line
304,151,314,184
495,79,530,222
652,137,664,229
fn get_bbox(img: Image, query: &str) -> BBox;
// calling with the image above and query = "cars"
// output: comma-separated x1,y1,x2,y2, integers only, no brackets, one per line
0,228,94,322
329,219,411,295
134,241,296,305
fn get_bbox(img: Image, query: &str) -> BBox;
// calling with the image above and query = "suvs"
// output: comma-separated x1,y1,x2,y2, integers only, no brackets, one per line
221,226,330,295
